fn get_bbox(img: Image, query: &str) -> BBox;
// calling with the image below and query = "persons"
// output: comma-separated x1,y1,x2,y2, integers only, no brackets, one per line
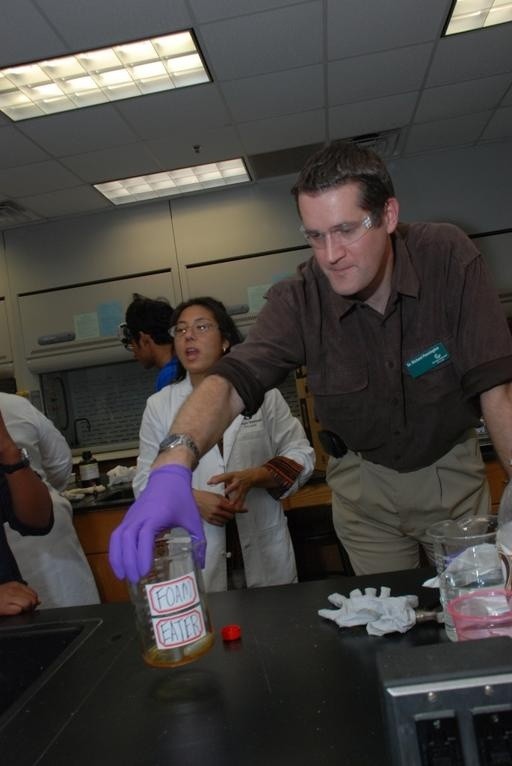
130,294,320,587
0,411,57,616
1,390,105,612
106,139,512,594
117,290,185,501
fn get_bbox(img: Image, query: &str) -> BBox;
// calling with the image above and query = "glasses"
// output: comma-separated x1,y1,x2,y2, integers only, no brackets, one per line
123,334,143,353
167,321,222,338
299,207,385,249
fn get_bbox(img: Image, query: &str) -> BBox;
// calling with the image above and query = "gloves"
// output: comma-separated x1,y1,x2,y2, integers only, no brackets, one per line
108,464,206,581
361,594,418,637
318,584,383,628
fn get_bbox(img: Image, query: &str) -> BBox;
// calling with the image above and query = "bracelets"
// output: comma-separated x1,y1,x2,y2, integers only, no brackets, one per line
1,445,34,474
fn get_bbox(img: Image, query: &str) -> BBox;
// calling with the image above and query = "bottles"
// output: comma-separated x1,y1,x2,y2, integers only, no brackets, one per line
79,452,100,487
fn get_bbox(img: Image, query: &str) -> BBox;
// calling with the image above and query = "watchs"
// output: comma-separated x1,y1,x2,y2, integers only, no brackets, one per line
155,433,203,469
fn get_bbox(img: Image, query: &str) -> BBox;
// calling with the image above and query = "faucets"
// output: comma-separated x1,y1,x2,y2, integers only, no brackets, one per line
72,417,92,445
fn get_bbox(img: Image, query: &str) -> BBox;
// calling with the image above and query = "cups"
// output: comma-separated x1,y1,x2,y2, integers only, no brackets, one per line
127,539,217,668
427,516,502,644
448,589,509,640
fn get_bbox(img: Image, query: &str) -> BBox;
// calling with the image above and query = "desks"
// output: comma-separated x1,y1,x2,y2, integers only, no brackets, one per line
70,440,512,603
